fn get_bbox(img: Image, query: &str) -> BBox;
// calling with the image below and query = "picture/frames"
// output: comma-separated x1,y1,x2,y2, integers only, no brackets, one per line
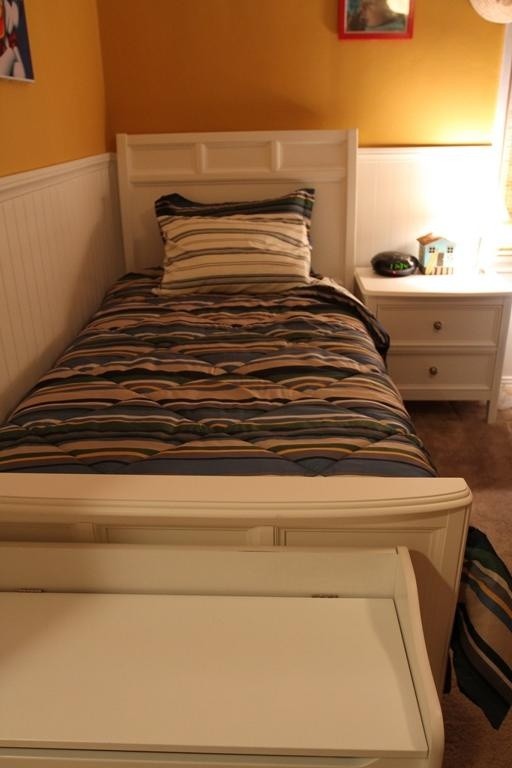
335,0,416,42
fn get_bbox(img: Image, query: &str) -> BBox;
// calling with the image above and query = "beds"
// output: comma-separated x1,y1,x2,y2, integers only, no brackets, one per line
1,126,474,699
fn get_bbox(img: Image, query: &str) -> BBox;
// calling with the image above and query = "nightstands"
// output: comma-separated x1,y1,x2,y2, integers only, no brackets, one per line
353,266,512,426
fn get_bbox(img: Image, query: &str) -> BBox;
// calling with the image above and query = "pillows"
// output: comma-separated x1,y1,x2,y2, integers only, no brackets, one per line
151,185,316,300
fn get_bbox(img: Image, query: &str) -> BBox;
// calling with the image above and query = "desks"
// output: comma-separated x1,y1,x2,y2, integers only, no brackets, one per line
2,544,449,768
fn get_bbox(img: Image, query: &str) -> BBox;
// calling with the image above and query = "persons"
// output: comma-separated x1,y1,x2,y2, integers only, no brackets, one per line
346,0,408,31
